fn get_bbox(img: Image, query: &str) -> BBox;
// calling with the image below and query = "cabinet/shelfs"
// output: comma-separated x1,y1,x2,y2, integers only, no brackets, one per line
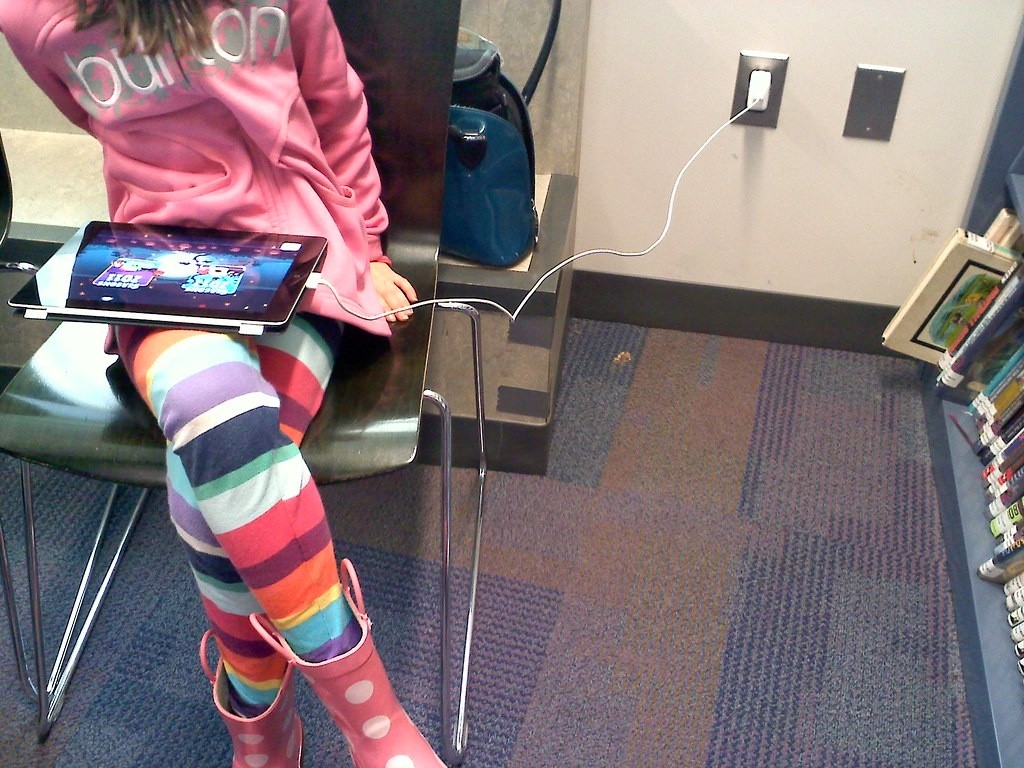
923,18,1024,768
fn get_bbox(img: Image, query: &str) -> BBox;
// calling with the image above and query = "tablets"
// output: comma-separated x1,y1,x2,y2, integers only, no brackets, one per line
7,220,328,328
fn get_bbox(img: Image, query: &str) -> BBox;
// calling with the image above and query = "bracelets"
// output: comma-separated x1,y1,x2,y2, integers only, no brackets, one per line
369,255,392,267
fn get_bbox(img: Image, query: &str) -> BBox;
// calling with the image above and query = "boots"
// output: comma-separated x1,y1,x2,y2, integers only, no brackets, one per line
249,558,452,768
200,628,302,768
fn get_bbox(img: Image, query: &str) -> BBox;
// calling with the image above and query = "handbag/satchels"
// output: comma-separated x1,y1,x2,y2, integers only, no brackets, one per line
439,27,539,267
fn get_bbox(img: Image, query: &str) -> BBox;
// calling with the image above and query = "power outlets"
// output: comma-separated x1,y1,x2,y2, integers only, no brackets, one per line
730,50,789,128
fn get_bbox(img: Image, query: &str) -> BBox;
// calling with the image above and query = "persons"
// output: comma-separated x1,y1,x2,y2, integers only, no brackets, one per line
0,0,453,768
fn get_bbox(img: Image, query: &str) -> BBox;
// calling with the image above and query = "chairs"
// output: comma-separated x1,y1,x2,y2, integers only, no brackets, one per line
0,0,487,767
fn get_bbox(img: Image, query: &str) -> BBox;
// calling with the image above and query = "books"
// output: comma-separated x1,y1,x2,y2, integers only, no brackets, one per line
881,209,1024,679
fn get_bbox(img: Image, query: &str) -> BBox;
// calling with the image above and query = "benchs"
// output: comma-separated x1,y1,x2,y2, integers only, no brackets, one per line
0,0,591,478
